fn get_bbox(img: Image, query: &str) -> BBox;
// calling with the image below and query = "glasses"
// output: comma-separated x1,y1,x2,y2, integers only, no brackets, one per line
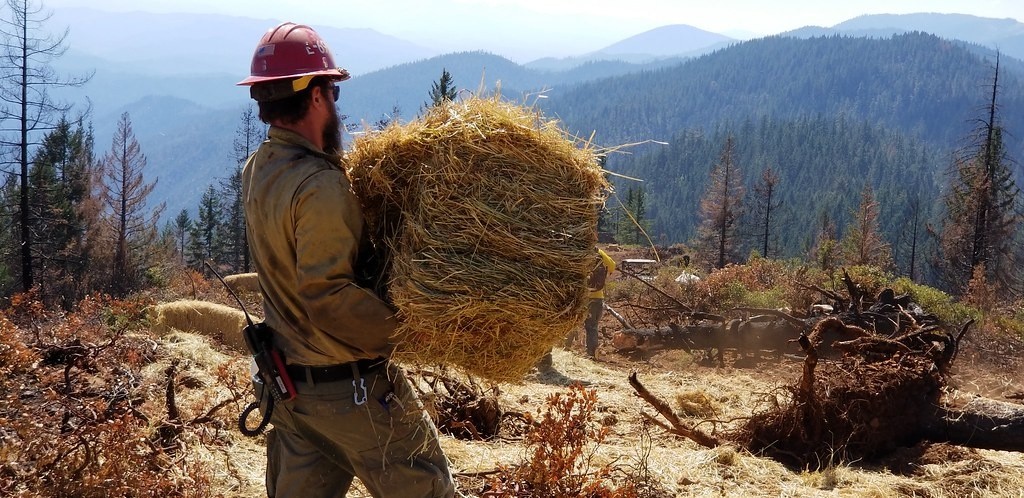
324,86,340,102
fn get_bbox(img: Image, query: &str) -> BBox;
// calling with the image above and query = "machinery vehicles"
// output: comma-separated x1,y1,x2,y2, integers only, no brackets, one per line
615,259,660,285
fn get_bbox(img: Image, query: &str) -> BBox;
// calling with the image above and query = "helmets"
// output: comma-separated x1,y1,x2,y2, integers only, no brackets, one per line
235,22,351,85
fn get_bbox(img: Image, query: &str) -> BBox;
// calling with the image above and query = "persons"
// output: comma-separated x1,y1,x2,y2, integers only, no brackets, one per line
587,247,608,358
234,21,462,498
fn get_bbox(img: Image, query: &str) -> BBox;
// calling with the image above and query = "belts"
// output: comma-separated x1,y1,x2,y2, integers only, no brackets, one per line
286,356,388,383
